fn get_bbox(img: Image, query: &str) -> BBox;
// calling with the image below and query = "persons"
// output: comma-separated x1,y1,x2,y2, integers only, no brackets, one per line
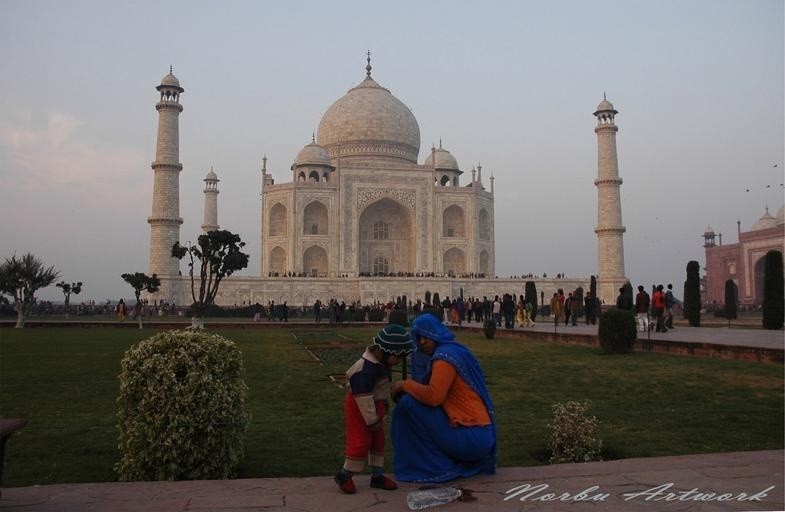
388,314,498,484
335,323,418,494
116,270,676,332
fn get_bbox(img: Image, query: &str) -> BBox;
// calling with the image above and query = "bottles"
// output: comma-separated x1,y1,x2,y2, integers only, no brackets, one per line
407,486,465,511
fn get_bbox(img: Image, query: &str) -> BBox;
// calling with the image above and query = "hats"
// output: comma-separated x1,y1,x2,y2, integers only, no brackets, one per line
373,324,417,355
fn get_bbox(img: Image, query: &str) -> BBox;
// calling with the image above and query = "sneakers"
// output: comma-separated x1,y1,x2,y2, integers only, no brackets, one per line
371,475,397,490
335,471,355,493
